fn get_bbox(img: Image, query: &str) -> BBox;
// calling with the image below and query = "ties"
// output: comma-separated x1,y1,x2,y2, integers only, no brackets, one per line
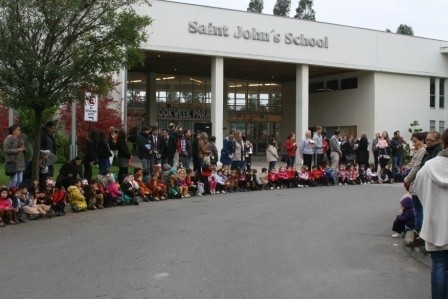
154,137,157,143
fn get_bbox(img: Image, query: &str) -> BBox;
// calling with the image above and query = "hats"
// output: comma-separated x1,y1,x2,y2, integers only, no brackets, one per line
39,149,50,154
81,179,88,185
109,126,114,132
399,197,414,208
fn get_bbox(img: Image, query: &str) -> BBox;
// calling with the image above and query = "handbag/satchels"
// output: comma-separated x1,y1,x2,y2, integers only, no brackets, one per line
203,156,210,165
403,181,410,192
4,160,17,176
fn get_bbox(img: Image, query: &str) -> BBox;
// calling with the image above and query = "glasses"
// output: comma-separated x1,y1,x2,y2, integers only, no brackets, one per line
425,138,439,142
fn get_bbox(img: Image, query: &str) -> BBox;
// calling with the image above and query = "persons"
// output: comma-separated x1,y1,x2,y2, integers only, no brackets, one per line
0,123,448,227
413,127,448,299
390,196,417,238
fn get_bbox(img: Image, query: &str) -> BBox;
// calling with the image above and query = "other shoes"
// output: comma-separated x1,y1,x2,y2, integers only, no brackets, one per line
148,196,154,201
185,194,191,197
306,185,309,188
160,196,165,200
221,190,226,194
405,238,425,247
338,183,342,186
271,186,273,190
134,201,139,205
392,233,403,238
277,186,280,190
298,184,301,188
344,183,348,186
144,198,149,202
154,196,159,201
0,222,5,227
360,179,376,185
300,184,304,188
217,191,220,194
8,220,16,224
